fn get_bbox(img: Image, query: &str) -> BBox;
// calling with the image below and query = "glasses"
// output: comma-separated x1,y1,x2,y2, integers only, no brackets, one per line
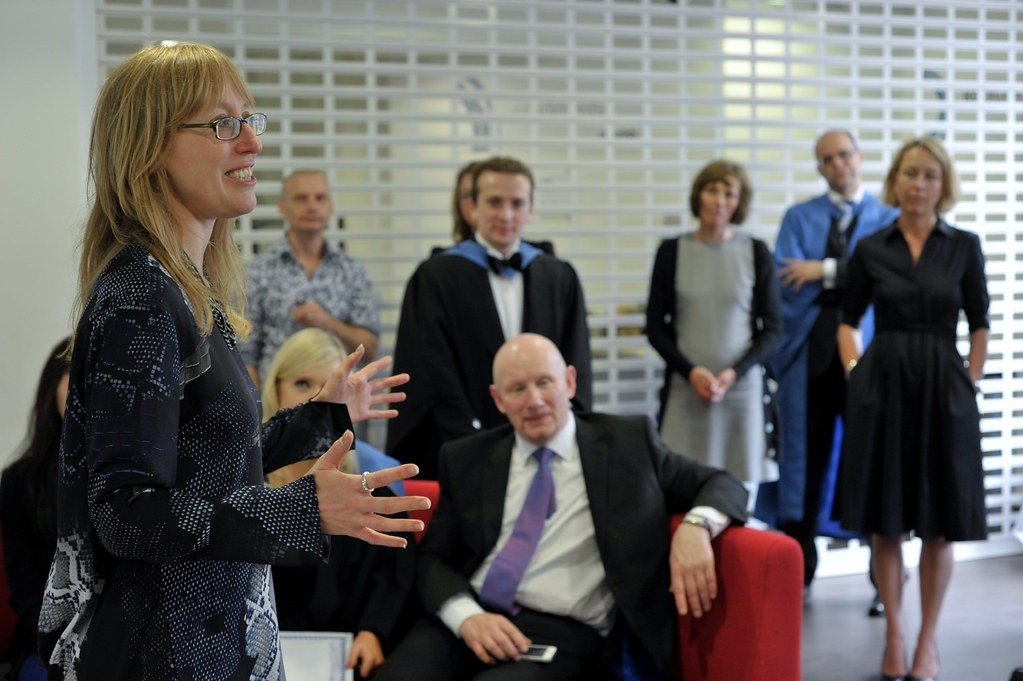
178,113,268,140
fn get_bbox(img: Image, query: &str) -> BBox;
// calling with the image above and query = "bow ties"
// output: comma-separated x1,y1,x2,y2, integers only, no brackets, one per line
484,251,525,276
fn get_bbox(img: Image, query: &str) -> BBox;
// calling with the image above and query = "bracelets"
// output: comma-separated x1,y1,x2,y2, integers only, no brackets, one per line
823,259,838,288
846,359,860,376
682,515,713,532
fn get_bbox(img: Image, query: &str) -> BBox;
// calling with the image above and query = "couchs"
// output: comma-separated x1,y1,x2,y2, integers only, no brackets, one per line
404,479,805,681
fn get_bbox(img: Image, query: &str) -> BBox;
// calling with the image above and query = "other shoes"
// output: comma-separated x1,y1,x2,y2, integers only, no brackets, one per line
870,593,887,615
908,655,941,681
882,644,908,681
804,586,812,607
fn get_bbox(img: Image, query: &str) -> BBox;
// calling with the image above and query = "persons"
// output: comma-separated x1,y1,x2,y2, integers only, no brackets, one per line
452,164,555,253
645,162,781,517
827,139,991,681
231,171,379,389
773,130,909,614
0,337,76,671
38,44,432,681
257,327,415,679
377,333,748,680
385,159,594,453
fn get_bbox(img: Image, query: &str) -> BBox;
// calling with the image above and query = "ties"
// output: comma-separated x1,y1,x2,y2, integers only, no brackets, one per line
478,446,557,616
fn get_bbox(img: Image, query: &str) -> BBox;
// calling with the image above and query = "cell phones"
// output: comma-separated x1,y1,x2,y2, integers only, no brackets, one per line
516,644,557,663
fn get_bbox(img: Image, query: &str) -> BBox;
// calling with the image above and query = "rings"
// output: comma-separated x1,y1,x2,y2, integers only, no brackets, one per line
362,472,374,490
